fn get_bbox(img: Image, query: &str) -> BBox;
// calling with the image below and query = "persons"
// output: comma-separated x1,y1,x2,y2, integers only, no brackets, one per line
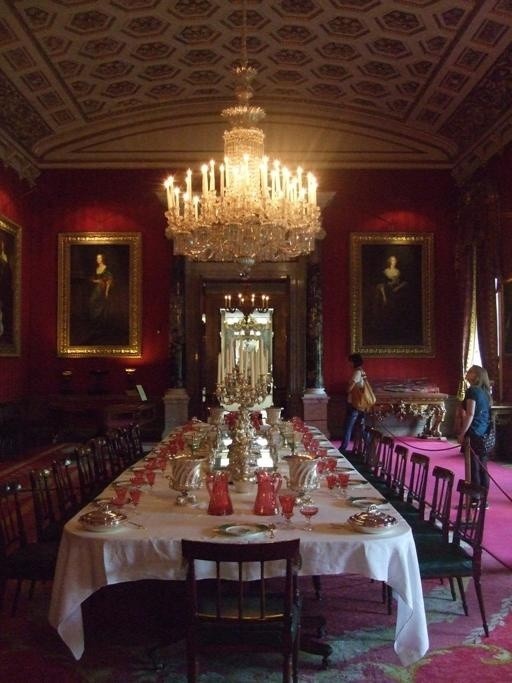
1,237,11,337
84,251,116,326
365,254,423,344
338,353,372,453
455,364,496,511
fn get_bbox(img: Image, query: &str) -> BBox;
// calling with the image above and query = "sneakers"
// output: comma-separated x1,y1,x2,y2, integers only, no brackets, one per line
463,502,478,508
477,504,489,511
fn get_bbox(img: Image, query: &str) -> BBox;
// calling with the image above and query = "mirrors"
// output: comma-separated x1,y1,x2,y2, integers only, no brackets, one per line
181,263,306,419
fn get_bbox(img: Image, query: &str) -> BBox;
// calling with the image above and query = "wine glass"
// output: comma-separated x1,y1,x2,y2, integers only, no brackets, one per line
301,498,318,532
115,419,195,523
291,416,349,498
280,488,297,529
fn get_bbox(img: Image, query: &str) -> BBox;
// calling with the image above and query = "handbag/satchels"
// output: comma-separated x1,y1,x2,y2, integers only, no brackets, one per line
351,378,377,409
461,423,496,455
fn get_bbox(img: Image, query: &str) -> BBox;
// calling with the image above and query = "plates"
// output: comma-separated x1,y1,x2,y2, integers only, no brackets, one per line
213,522,268,537
305,427,395,535
77,427,181,532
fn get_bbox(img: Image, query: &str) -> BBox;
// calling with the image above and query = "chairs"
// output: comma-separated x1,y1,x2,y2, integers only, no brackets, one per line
340,424,491,639
0,416,153,617
180,539,308,683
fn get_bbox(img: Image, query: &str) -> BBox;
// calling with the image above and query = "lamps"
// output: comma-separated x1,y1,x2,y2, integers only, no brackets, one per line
221,283,270,327
161,3,328,281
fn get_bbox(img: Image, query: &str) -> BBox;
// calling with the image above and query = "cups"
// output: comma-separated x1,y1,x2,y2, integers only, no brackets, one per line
206,471,233,515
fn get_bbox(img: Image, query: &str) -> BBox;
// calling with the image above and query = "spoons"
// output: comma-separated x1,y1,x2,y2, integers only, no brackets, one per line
269,523,275,536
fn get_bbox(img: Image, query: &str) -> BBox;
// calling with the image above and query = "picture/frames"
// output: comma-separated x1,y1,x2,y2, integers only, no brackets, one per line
0,215,28,360
347,231,434,359
54,229,146,360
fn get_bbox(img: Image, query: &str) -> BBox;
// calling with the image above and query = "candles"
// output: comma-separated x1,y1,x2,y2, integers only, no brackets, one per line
216,335,265,387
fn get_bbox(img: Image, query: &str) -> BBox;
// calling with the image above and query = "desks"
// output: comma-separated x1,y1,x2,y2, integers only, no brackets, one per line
371,391,449,440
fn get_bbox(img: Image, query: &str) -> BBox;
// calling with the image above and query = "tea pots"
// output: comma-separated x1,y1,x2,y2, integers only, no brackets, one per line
255,472,284,515
